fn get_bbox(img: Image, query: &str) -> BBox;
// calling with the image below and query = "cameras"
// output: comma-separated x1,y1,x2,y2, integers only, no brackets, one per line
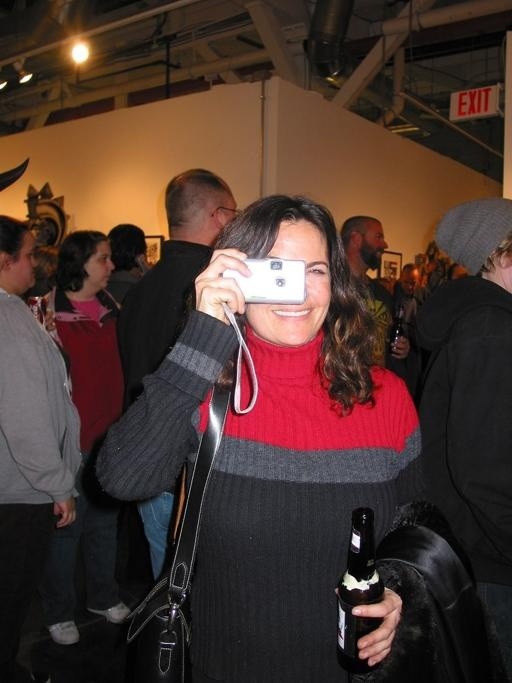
221,260,305,305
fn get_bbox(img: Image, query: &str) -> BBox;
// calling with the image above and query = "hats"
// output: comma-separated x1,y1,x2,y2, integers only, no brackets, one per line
435,198,512,276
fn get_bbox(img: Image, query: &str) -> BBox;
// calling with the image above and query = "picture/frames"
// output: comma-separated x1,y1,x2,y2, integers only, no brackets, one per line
144,234,164,266
377,250,404,280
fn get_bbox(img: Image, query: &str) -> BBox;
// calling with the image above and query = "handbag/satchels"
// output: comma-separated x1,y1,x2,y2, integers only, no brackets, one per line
126,574,192,683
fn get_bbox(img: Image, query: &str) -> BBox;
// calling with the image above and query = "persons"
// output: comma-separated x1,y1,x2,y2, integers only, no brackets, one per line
0,169,512,683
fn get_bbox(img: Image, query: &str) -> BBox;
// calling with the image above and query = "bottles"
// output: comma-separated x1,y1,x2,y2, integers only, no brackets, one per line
333,508,385,675
388,302,405,353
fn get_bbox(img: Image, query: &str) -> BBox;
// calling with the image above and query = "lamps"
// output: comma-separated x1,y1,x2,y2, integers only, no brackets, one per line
12,54,33,84
0,77,8,89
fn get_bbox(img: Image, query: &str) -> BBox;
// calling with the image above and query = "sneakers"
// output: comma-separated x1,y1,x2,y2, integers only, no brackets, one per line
87,602,131,625
44,621,80,645
